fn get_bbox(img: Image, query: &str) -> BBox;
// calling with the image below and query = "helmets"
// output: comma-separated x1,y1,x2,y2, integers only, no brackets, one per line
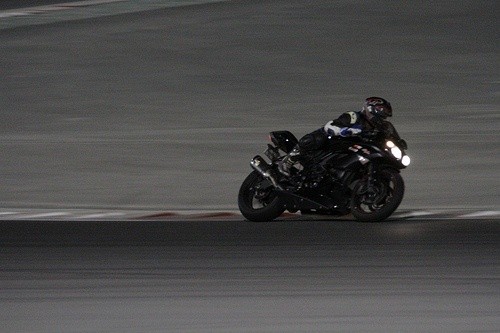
363,97,392,117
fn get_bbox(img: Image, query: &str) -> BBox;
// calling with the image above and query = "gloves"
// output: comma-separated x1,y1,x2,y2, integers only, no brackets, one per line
340,127,360,137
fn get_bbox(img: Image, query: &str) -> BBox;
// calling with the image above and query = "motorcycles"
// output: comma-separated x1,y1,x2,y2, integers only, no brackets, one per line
238,121,411,222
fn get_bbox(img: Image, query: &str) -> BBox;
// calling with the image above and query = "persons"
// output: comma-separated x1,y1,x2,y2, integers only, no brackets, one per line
301,96,394,189
